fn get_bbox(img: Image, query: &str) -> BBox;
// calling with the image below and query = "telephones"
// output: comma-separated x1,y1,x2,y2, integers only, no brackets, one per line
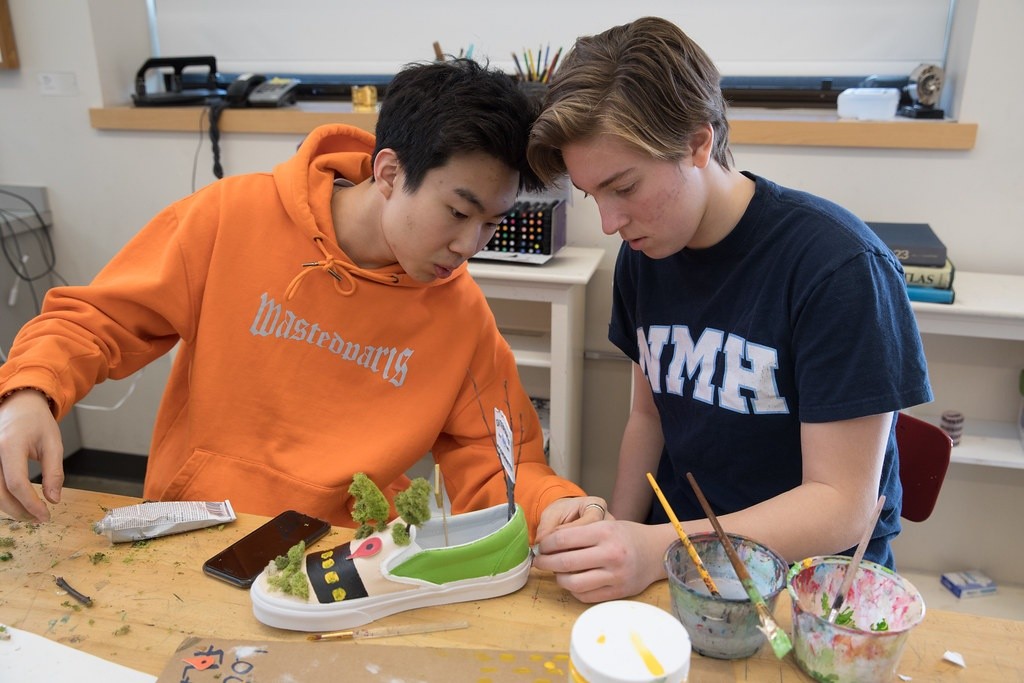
227,72,302,108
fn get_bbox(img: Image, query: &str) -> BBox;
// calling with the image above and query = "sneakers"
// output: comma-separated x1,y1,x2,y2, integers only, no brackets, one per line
250,503,531,632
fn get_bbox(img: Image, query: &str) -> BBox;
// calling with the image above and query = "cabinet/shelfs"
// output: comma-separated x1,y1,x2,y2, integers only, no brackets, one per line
909,271,1024,471
465,247,607,486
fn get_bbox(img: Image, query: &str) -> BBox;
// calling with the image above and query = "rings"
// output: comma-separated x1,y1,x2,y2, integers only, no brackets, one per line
582,503,605,520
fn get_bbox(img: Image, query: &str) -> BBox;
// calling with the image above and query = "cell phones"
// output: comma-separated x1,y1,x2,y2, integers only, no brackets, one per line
203,510,331,589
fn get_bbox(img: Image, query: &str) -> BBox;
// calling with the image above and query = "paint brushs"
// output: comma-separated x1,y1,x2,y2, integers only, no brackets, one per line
460,48,464,59
646,470,721,599
824,495,887,625
510,41,563,82
685,469,794,661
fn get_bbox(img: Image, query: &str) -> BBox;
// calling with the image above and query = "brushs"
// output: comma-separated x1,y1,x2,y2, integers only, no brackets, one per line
432,40,444,60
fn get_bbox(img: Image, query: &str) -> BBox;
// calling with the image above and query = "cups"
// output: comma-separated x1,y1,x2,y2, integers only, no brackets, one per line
786,556,925,683
568,600,691,683
663,532,790,660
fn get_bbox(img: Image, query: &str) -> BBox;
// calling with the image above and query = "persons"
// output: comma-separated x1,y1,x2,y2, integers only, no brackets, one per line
0,55,619,549
523,15,934,605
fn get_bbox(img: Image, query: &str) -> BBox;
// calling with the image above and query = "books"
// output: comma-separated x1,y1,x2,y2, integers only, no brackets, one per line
858,222,956,305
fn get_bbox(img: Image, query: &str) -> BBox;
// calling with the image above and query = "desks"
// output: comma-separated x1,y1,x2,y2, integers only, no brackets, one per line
0,481,1024,683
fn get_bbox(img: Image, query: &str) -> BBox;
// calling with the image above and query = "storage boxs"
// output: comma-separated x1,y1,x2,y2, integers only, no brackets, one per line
943,570,998,599
